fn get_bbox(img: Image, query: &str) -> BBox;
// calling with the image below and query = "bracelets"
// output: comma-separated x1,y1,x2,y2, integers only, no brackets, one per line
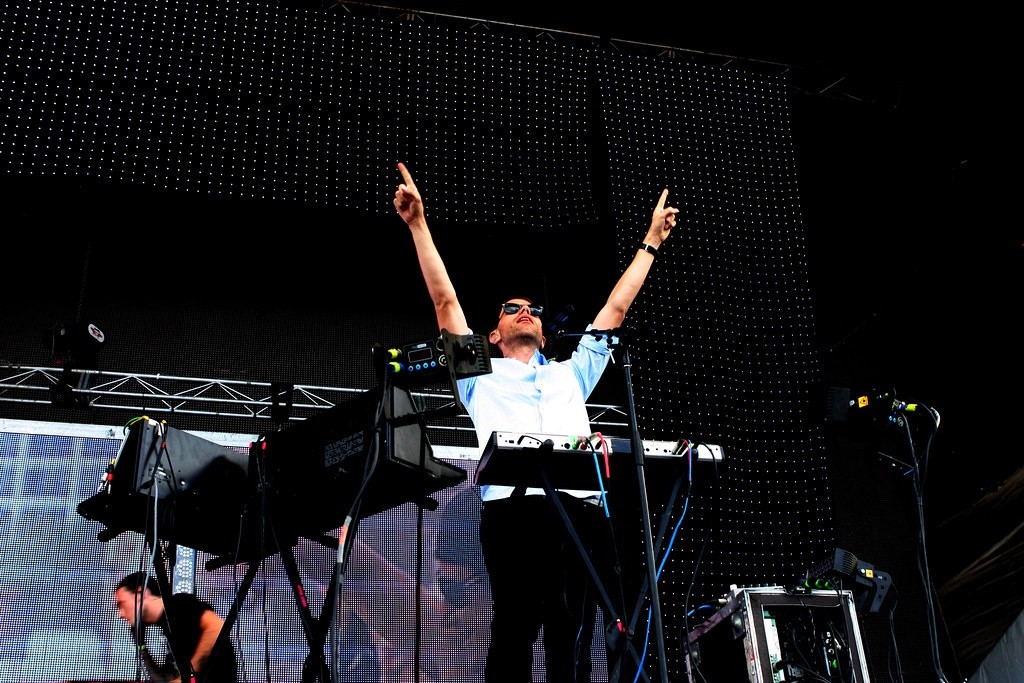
638,243,659,258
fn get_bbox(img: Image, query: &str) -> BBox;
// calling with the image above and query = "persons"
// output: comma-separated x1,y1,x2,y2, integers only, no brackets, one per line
393,162,679,683
115,572,238,683
293,485,548,683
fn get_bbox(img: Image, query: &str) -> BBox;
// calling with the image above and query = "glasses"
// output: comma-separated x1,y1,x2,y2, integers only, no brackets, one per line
499,303,543,323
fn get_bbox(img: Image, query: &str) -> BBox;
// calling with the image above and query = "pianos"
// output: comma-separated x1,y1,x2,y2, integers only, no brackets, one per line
474,430,723,498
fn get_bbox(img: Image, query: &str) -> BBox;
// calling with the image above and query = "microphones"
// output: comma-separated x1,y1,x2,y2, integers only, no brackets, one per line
548,305,574,332
850,386,896,409
